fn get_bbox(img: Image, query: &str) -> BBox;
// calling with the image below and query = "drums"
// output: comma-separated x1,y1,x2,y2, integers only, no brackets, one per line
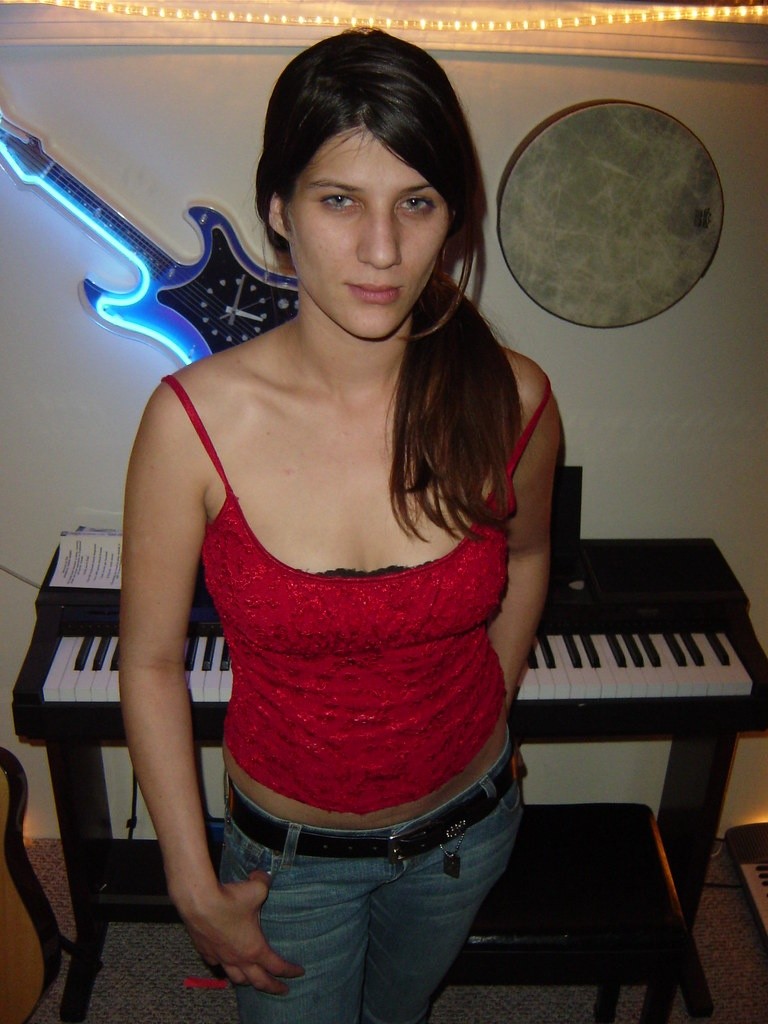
496,99,725,330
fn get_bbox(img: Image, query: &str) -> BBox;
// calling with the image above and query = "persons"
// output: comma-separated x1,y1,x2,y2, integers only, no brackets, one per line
118,31,562,1023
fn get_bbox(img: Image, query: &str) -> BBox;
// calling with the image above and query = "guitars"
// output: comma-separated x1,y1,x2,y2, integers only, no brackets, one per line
0,113,298,370
0,747,63,1024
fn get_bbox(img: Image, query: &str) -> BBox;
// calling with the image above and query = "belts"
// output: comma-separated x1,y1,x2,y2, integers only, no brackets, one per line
228,748,521,861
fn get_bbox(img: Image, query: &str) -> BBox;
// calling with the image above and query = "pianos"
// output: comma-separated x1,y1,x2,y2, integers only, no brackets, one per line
10,530,767,746
